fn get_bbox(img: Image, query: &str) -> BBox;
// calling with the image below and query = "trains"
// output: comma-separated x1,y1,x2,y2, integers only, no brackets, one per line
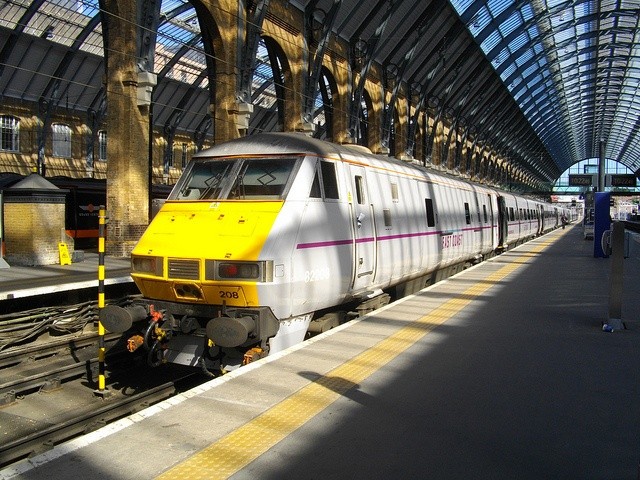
0,171,178,251
129,131,583,379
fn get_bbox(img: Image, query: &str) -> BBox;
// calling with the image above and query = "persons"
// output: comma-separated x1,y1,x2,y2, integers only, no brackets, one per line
561,214,568,228
